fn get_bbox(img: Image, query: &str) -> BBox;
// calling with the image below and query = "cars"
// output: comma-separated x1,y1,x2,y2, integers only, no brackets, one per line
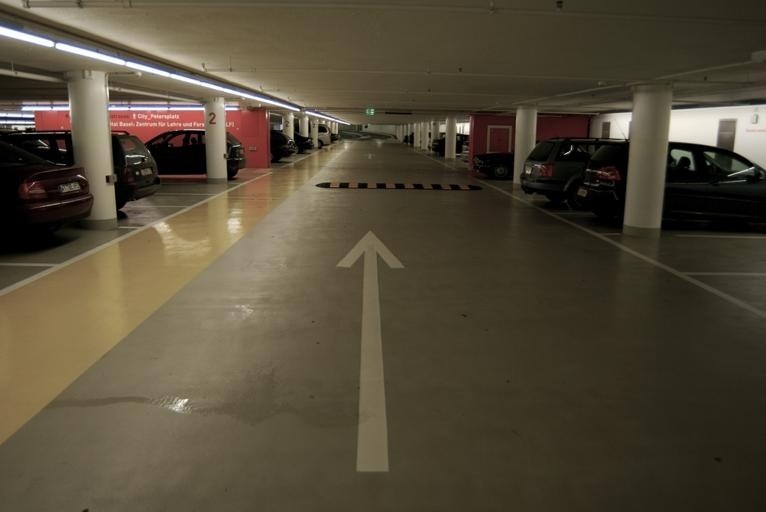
472,140,540,180
431,131,469,162
144,129,247,179
0,130,160,228
270,124,339,162
520,137,766,227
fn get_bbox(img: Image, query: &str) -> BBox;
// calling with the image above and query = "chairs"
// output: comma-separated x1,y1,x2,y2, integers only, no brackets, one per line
190,137,197,144
667,155,691,182
